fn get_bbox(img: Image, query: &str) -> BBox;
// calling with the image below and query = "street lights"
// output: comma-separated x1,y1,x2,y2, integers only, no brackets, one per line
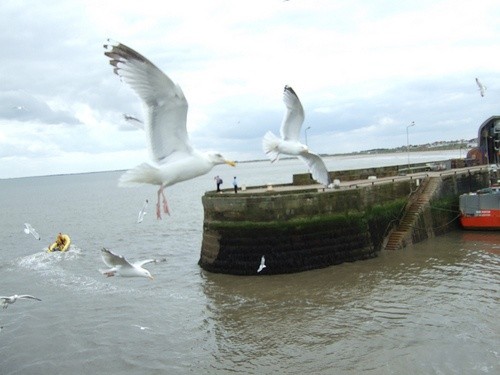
406,122,416,172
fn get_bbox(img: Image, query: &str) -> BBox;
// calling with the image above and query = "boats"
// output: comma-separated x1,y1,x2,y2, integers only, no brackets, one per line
458,181,500,231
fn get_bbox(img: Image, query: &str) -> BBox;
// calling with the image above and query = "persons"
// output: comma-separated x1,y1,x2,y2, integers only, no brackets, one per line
214,175,221,192
55,232,65,246
233,176,239,194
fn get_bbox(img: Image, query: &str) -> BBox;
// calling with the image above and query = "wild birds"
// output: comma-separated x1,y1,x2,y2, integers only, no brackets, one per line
23,222,42,241
99,32,240,221
474,76,489,99
256,255,267,272
261,83,331,189
0,293,44,310
95,245,168,281
137,199,150,224
